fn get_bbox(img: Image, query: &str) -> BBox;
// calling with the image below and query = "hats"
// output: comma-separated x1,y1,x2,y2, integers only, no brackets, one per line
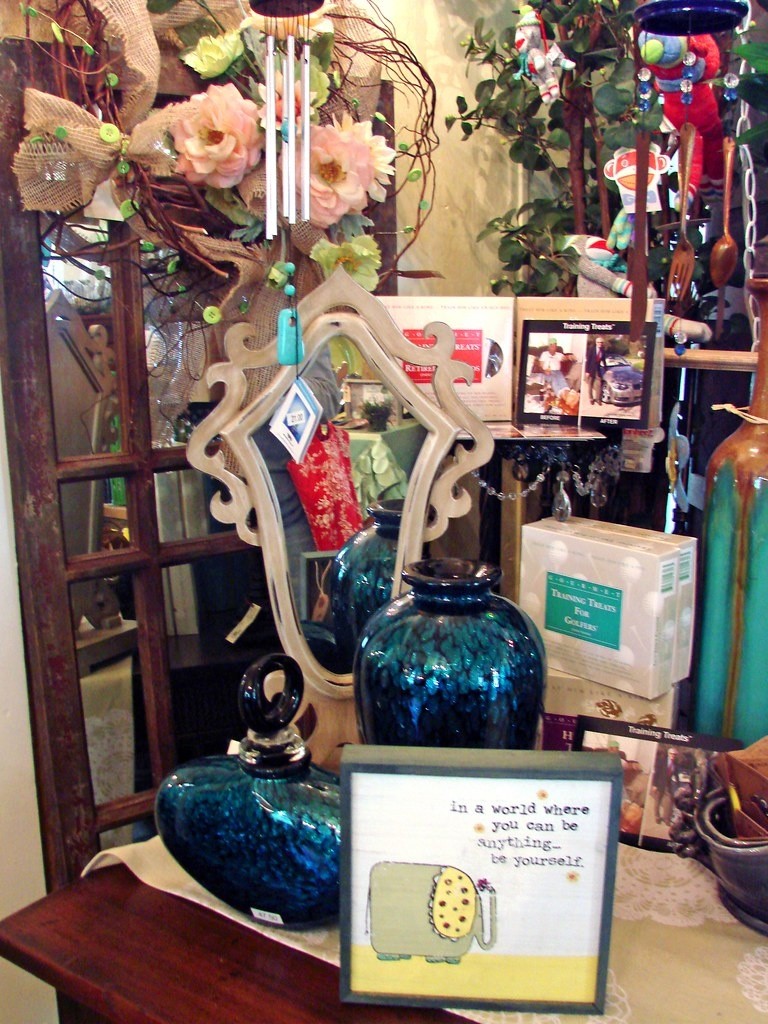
549,338,557,344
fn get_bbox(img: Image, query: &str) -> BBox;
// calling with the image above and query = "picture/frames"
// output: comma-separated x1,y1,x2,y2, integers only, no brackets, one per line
515,319,661,432
571,716,744,859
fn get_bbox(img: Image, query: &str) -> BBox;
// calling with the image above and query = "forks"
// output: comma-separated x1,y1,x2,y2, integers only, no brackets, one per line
666,123,695,302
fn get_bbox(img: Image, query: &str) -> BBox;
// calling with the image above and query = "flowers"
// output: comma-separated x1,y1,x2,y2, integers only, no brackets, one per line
147,0,396,291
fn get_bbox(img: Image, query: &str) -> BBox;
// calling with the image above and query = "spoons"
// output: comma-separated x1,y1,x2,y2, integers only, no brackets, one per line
710,137,738,288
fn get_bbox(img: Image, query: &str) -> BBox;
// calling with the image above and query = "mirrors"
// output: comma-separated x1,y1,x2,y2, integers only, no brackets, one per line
185,263,495,772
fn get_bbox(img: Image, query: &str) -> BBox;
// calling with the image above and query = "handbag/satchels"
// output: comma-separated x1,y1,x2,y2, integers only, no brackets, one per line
285,422,364,551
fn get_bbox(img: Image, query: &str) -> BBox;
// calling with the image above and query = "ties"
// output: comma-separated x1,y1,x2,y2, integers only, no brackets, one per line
597,347,601,361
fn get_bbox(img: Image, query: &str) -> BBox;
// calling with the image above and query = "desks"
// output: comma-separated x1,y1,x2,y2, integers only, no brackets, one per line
0,843,768,1024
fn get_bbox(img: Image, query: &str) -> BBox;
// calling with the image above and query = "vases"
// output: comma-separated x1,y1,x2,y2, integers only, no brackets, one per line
330,499,404,674
353,558,546,751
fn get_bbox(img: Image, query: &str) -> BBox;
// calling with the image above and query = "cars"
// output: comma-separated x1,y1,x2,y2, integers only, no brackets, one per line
584,352,642,404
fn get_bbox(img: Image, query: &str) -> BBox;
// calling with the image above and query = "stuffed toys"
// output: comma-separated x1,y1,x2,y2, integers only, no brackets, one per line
573,232,711,343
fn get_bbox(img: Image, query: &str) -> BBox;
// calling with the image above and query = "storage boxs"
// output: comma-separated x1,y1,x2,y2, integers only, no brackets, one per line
520,516,698,729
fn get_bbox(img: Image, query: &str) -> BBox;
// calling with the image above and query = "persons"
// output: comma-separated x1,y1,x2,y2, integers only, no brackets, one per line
538,337,576,397
585,337,606,406
654,748,679,826
250,341,344,623
609,740,639,804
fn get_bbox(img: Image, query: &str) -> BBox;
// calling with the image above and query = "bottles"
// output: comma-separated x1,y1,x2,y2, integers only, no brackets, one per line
151,654,341,934
303,498,404,676
352,556,544,750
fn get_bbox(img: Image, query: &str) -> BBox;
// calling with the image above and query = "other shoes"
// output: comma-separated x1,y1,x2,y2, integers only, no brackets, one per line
590,399,595,405
560,387,569,401
597,399,602,405
